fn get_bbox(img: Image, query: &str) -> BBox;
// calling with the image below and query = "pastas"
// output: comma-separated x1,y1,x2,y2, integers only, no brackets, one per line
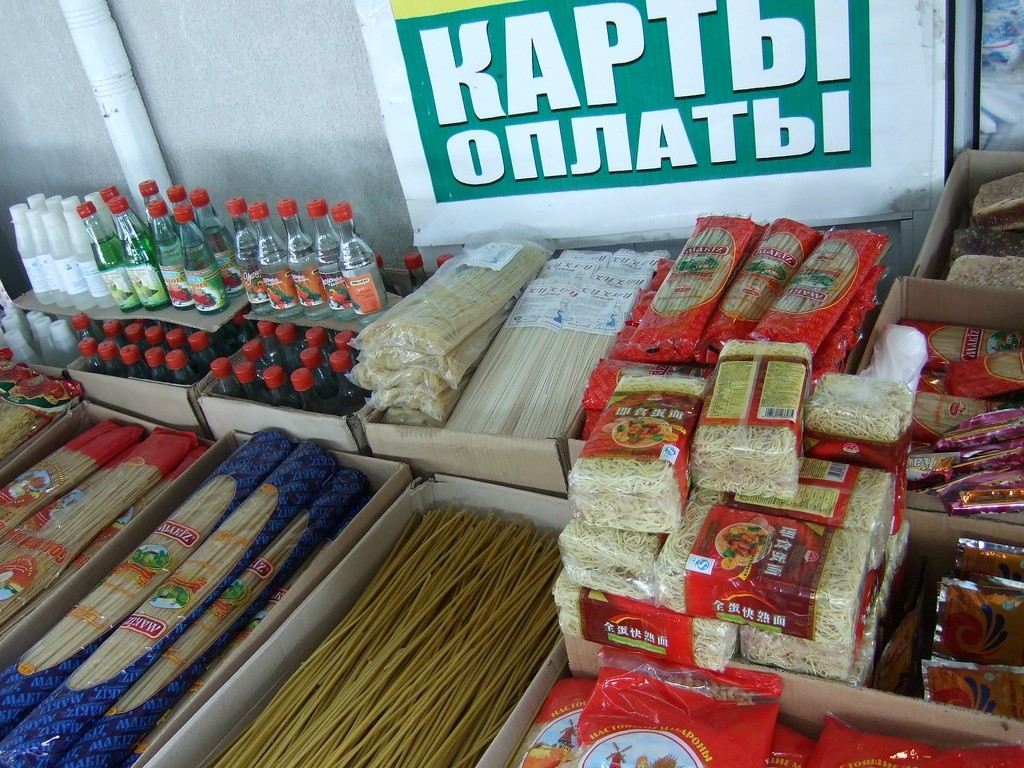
551,214,1024,681
0,348,570,768
347,238,672,449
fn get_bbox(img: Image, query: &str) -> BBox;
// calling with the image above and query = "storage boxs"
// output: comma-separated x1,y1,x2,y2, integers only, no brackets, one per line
0,147,1024,768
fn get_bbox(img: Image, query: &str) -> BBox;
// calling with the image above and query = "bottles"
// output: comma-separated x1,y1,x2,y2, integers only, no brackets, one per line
72,306,258,384
373,251,403,300
404,252,428,293
75,179,246,315
275,198,332,322
306,198,357,321
437,254,454,268
0,305,81,365
246,201,304,320
225,197,273,315
210,321,372,415
329,202,390,327
10,191,129,310
